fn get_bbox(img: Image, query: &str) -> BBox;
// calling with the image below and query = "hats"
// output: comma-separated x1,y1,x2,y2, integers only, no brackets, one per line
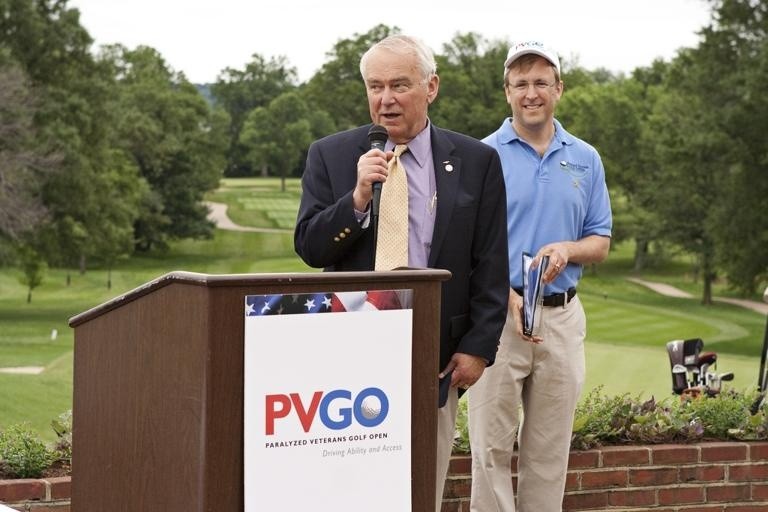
503,40,561,80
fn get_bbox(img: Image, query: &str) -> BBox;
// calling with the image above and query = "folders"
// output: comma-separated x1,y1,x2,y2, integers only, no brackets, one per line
520,251,549,336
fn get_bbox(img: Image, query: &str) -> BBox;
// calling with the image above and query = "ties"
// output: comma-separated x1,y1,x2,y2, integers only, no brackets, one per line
374,145,408,271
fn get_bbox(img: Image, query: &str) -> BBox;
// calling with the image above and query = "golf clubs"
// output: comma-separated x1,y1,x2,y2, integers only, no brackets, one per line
666,337,734,389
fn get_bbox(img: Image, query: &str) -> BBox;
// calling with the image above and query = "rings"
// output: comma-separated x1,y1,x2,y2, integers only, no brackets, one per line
555,264,561,272
462,384,470,390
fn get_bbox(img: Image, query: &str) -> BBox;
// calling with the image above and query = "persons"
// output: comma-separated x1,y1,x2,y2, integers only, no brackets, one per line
292,31,512,512
465,38,618,512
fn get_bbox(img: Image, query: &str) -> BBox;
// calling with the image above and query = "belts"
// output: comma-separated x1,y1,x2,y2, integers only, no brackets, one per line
512,287,576,307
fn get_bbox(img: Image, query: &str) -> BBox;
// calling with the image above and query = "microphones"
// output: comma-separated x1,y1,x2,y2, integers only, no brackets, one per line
367,124,388,191
